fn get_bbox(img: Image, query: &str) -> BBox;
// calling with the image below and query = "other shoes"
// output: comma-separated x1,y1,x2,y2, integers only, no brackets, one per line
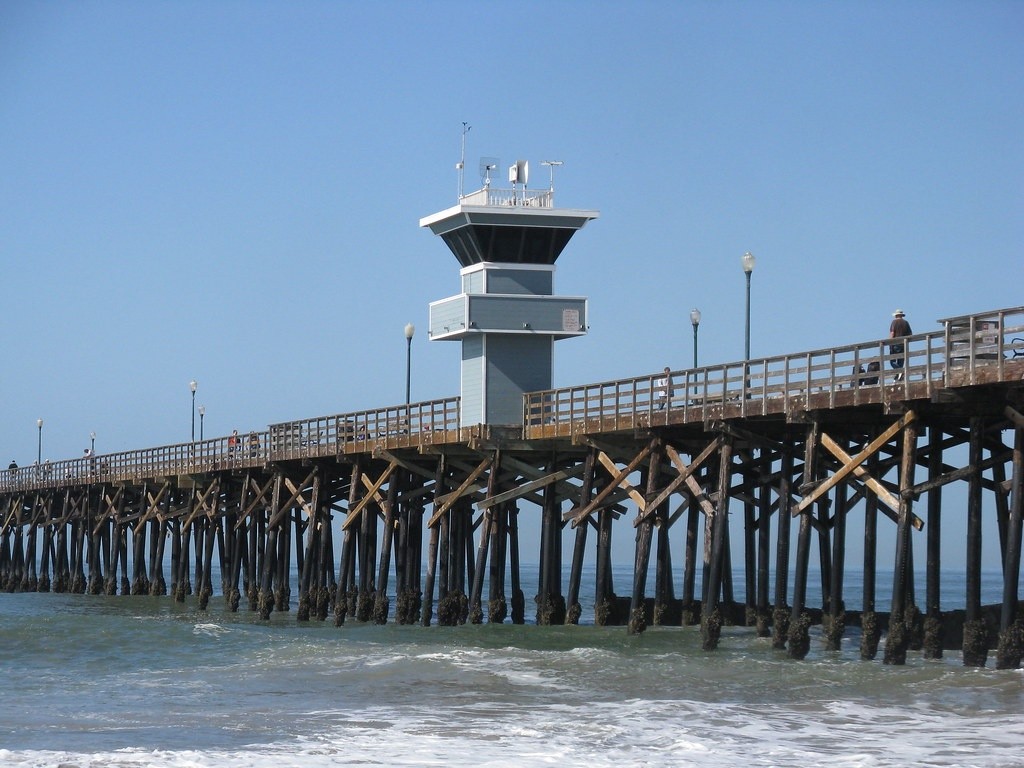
893,379,899,383
898,372,904,381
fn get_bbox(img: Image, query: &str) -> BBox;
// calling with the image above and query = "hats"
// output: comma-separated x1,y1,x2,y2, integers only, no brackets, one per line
892,310,905,316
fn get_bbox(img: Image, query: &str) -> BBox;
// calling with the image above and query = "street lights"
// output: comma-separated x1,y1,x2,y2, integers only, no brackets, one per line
90,431,96,475
197,404,205,465
37,418,43,464
404,322,415,434
190,379,197,465
689,307,702,406
739,251,757,399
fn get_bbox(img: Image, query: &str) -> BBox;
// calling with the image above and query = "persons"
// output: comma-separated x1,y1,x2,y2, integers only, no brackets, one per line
658,367,674,410
8,449,109,485
357,425,370,440
227,430,261,459
850,309,913,388
